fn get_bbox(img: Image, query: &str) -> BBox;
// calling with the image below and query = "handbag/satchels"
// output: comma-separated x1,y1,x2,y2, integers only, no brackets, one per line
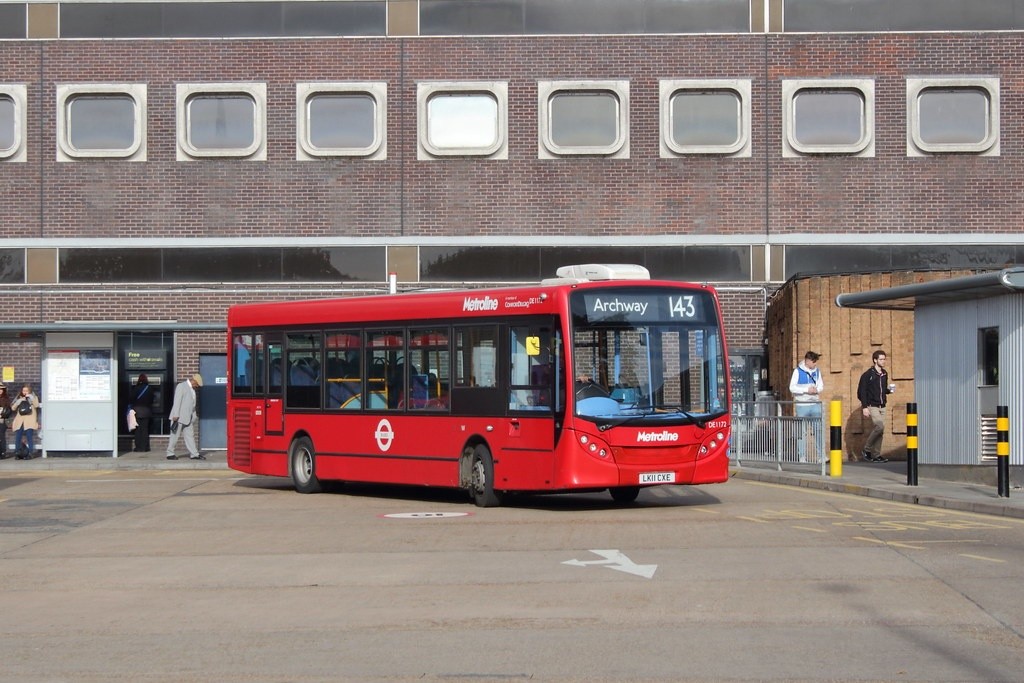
127,409,138,432
0,405,12,419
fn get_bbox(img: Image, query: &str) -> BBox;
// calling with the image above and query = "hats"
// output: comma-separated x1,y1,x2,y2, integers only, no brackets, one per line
0,382,5,388
193,373,204,386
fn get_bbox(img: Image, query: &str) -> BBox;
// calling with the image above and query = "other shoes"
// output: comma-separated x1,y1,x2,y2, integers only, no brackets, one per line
190,454,206,460
1,454,5,459
818,457,830,463
799,457,806,463
15,455,20,459
167,455,178,460
134,448,147,452
23,455,31,460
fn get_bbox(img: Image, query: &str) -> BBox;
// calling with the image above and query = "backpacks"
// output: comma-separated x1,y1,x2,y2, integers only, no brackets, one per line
19,397,32,415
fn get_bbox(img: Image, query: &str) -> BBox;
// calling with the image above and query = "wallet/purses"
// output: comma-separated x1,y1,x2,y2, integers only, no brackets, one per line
171,421,178,432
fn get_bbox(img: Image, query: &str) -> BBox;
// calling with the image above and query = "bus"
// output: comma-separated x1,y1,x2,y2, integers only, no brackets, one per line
226,262,732,508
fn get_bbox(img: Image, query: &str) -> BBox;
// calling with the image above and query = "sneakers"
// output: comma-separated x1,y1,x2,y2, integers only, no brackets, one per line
873,456,888,463
861,450,873,462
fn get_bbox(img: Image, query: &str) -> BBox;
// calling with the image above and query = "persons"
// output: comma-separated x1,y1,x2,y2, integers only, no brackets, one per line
0,382,11,459
10,383,39,460
128,373,154,452
166,374,206,460
789,351,830,464
858,350,896,463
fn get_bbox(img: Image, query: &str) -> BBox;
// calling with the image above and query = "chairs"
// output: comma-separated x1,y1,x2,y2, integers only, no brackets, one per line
317,357,351,381
397,357,418,392
368,357,386,390
337,373,361,408
244,358,263,386
298,356,320,377
290,358,317,385
270,358,293,386
